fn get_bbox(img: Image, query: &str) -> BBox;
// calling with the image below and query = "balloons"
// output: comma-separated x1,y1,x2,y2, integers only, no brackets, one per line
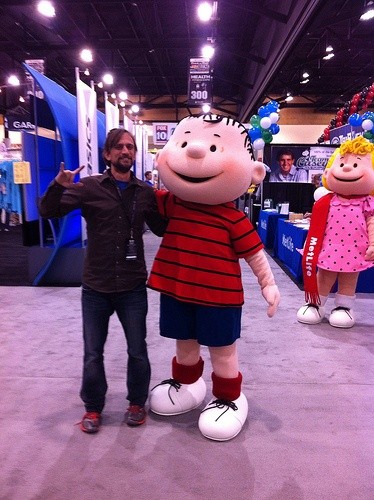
317,83,374,143
247,100,280,151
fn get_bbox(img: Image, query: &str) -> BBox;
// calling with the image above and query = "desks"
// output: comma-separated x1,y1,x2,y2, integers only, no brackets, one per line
258,209,289,249
276,219,374,292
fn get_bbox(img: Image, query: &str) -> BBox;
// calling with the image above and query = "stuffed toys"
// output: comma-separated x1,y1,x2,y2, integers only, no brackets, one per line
297,137,374,329
145,112,281,442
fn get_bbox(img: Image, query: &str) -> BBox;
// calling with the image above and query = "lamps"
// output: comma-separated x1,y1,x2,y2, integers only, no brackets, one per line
321,34,334,60
300,69,310,84
359,0,374,21
285,89,293,102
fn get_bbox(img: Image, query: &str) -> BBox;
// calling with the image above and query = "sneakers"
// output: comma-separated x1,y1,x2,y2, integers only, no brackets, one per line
80,412,102,432
124,405,146,424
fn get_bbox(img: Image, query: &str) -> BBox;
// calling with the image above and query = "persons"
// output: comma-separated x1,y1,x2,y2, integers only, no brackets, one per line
270,149,308,183
40,129,167,434
145,171,155,232
302,173,322,220
